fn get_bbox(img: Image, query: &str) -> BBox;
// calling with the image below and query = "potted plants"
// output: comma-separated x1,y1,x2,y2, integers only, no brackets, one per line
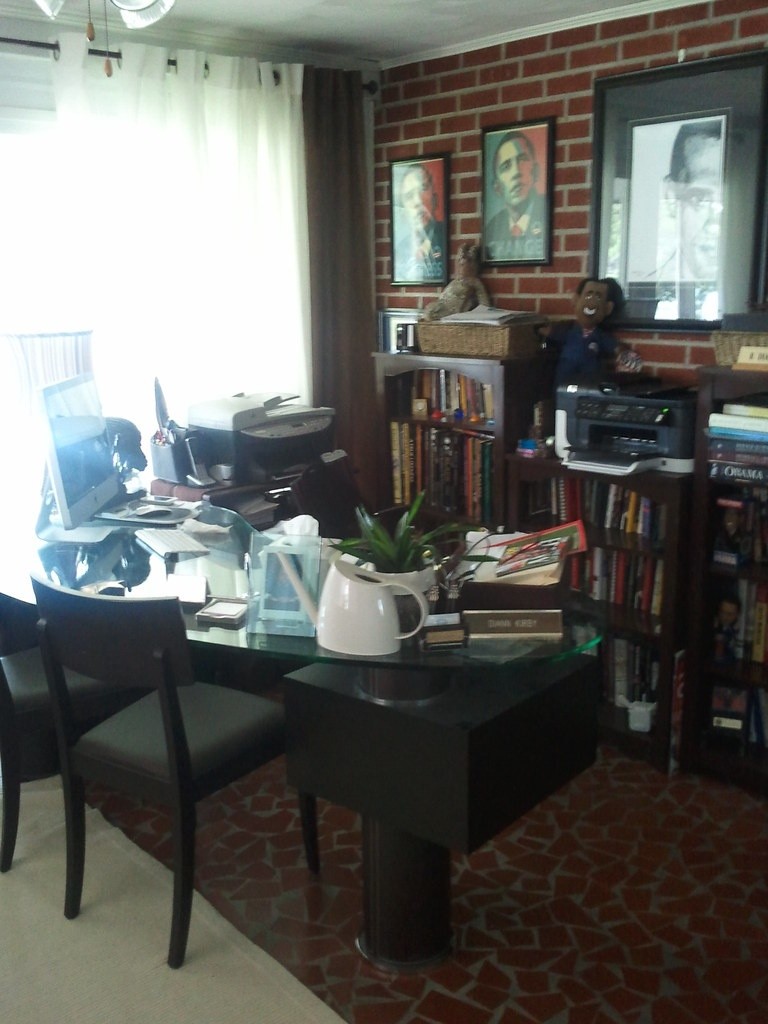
337,489,502,596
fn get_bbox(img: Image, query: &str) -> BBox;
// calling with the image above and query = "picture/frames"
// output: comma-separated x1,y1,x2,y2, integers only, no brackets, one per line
591,47,767,331
480,115,552,265
389,150,454,287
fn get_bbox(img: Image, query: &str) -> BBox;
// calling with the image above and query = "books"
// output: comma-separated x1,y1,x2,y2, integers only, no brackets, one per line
680,475,768,747
494,477,666,717
389,368,494,526
706,390,768,482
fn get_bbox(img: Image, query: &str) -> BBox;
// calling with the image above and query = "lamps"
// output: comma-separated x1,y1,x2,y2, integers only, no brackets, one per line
35,0,176,79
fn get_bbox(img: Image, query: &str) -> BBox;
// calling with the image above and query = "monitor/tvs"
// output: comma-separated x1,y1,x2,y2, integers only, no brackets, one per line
35,373,118,542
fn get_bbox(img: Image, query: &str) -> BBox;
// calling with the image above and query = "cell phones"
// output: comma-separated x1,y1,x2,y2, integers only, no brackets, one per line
184,437,208,480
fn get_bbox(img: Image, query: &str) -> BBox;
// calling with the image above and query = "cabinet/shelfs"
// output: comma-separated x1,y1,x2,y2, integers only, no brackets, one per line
373,351,768,804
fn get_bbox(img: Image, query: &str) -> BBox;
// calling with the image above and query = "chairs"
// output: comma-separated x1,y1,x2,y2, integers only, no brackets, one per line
32,575,318,968
0,657,106,869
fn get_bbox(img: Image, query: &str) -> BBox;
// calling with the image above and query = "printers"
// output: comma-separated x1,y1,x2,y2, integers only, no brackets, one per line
189,392,350,483
553,373,698,478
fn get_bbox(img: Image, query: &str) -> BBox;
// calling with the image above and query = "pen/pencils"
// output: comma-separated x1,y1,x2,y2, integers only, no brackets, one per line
244,553,253,595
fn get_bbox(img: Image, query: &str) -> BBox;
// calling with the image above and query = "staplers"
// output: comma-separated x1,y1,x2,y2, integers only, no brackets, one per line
209,463,235,487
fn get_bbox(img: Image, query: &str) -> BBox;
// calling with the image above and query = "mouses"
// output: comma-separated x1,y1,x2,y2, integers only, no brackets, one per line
136,505,171,517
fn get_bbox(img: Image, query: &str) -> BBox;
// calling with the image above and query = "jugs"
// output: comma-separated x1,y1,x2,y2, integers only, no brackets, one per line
277,552,429,655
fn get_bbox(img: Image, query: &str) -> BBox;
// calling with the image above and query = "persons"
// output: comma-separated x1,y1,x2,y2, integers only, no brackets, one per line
396,163,443,281
484,131,545,261
716,507,745,553
702,594,743,655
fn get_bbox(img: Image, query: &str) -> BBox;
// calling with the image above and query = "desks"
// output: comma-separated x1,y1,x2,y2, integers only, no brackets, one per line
0,484,613,970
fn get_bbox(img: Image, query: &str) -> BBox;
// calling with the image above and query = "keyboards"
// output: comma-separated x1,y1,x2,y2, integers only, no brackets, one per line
135,530,210,558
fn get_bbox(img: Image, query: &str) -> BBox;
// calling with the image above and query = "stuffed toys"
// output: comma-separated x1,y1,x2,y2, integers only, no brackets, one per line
535,277,643,394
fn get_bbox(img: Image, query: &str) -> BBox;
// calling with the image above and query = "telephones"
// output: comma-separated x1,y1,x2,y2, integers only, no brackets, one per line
184,435,217,488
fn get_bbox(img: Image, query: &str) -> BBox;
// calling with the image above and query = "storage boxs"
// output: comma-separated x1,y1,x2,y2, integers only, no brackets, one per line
414,319,549,357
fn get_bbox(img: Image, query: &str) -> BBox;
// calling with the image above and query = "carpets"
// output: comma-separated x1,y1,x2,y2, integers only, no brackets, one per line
0,771,347,1023
